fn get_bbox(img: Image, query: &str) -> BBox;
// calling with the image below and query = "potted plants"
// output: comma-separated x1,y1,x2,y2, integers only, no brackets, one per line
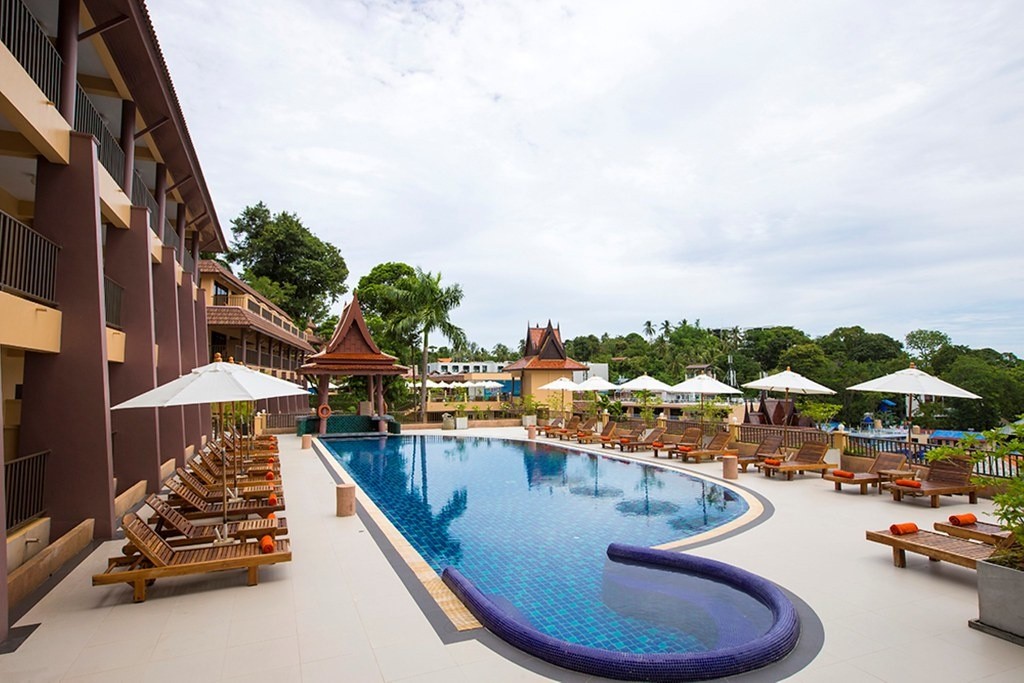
538,408,548,426
520,395,537,426
455,404,468,429
928,414,1024,650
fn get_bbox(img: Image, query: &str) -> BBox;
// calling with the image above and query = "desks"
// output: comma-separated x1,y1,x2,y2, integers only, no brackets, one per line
578,429,593,446
877,469,915,499
758,452,786,475
620,435,638,453
674,442,697,458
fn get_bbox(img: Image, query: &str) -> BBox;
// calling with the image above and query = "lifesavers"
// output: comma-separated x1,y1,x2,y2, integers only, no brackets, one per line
383,401,387,415
317,404,331,419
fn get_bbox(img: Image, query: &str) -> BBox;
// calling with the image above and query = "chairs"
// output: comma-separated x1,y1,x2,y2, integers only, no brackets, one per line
824,453,905,496
933,519,1014,546
681,430,738,463
865,515,1024,573
882,454,978,508
525,415,564,436
601,426,646,451
616,426,668,452
554,417,597,440
547,415,580,438
578,421,618,444
718,436,786,474
92,429,291,603
765,441,837,481
651,427,702,459
